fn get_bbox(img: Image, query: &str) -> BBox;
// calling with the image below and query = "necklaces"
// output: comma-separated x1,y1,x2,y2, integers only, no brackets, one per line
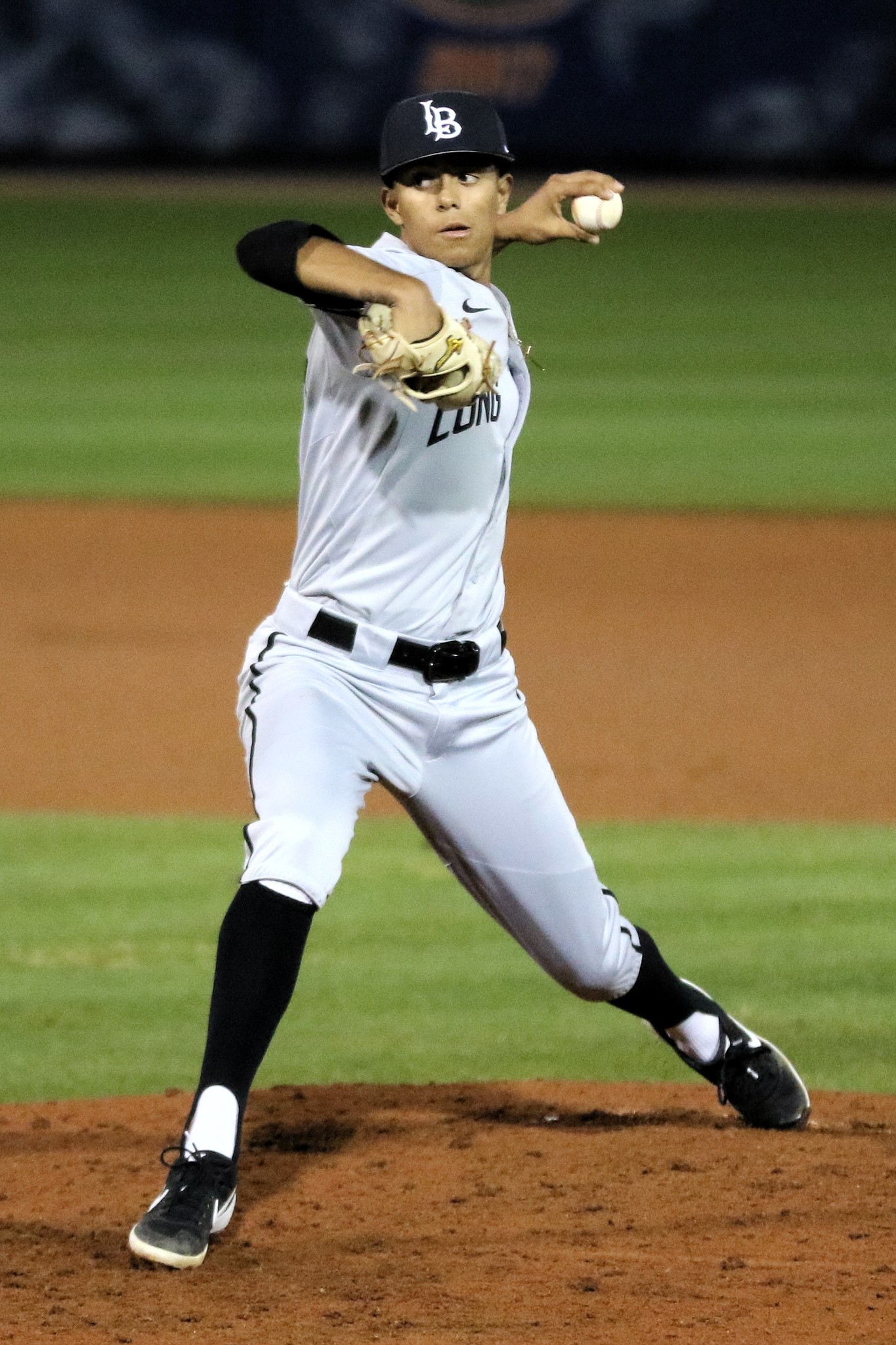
508,329,546,371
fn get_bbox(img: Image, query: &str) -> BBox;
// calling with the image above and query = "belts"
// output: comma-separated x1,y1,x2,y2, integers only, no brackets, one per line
310,610,510,684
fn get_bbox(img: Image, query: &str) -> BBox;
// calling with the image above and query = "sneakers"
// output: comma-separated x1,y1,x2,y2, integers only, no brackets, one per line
127,1141,237,1270
645,977,813,1128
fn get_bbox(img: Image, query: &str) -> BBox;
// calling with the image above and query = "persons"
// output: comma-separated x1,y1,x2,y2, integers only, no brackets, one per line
128,90,814,1270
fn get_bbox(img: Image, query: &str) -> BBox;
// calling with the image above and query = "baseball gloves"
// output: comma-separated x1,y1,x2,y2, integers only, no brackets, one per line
353,299,505,417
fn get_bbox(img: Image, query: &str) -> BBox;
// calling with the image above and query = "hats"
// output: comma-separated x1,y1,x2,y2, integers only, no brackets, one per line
379,92,516,183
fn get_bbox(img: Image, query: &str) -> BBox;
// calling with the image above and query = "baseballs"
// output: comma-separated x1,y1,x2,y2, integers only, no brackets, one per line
570,189,625,233
